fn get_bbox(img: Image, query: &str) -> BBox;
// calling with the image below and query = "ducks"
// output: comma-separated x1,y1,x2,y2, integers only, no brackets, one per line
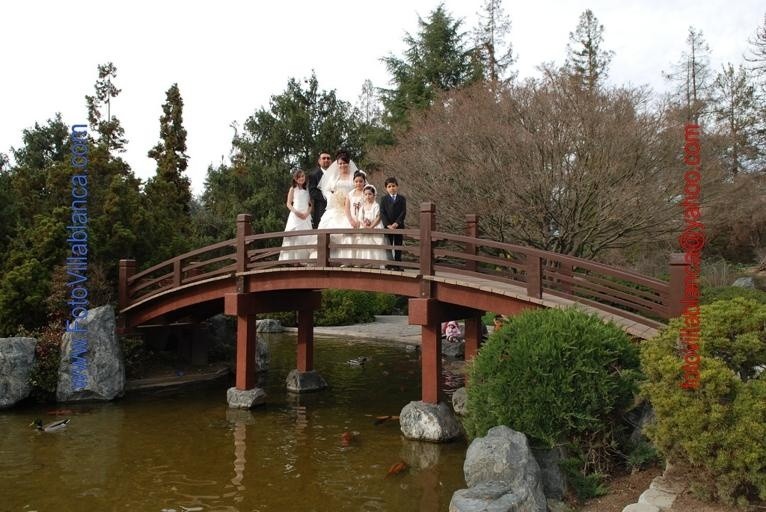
28,417,72,433
345,354,370,367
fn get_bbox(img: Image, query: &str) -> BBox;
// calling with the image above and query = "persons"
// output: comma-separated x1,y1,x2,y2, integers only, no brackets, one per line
278,169,314,267
308,151,368,268
339,170,367,268
380,176,406,273
309,151,332,229
445,320,460,343
353,184,386,270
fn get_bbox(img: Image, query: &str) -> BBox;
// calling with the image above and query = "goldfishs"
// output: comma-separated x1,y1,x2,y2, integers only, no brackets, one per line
342,429,360,449
365,414,401,422
48,407,92,415
384,460,411,479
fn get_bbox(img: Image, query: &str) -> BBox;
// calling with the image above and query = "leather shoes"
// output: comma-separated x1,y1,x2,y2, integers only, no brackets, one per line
340,263,405,272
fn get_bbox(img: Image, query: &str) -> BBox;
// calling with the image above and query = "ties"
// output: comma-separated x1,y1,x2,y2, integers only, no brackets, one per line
392,195,395,202
